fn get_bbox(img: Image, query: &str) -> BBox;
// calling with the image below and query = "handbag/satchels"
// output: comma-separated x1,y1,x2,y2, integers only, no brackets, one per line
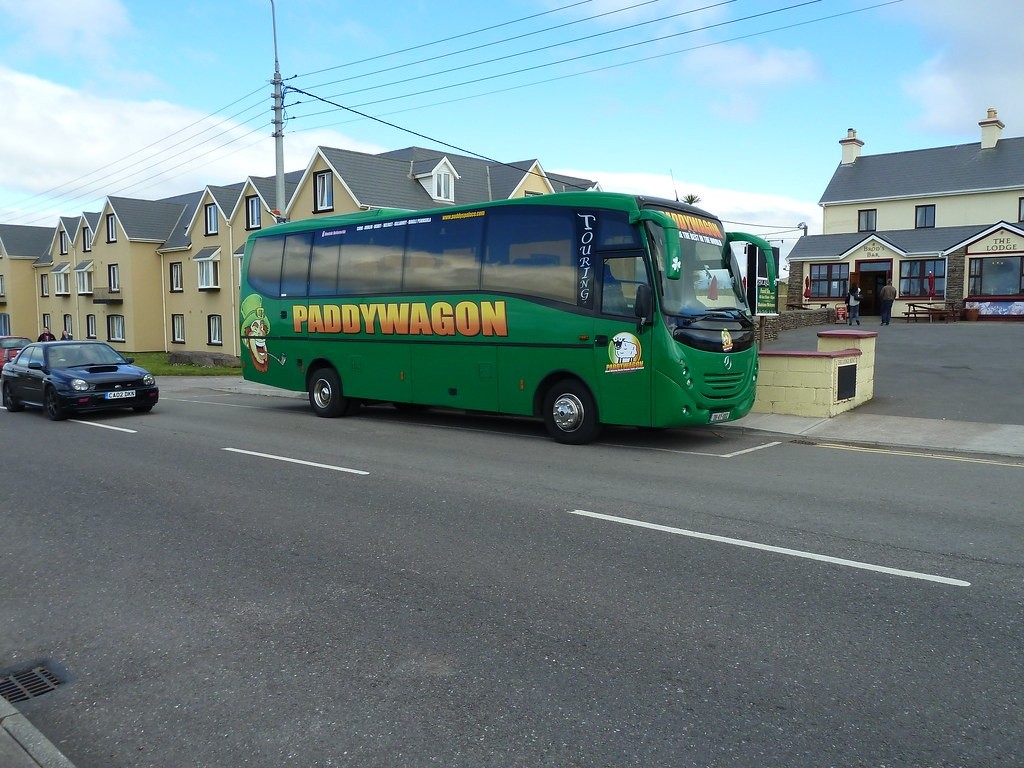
854,294,864,301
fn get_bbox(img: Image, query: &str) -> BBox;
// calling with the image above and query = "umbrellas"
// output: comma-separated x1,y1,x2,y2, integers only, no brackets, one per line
804,275,811,308
928,270,935,323
743,276,746,292
707,275,717,301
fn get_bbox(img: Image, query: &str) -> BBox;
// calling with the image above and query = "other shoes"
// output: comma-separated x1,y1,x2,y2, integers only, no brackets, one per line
849,323,852,325
881,320,886,324
856,320,860,325
886,322,889,325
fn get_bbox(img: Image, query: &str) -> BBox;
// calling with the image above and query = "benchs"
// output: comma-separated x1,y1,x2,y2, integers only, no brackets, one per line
902,311,951,315
911,308,960,313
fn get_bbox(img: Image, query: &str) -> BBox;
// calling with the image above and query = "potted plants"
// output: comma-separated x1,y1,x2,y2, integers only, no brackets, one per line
963,308,979,321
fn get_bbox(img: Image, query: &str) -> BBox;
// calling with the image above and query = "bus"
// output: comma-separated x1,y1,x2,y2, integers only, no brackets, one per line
237,189,777,446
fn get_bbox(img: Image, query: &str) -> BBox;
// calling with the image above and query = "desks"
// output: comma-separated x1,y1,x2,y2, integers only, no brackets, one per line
905,302,956,324
785,303,829,310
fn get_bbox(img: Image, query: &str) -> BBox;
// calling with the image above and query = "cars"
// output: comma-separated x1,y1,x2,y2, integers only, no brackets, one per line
0,340,160,422
0,334,35,376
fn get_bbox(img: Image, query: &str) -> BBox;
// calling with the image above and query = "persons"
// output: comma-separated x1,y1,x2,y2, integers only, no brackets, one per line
60,330,71,340
879,279,897,326
37,327,56,342
845,282,861,326
